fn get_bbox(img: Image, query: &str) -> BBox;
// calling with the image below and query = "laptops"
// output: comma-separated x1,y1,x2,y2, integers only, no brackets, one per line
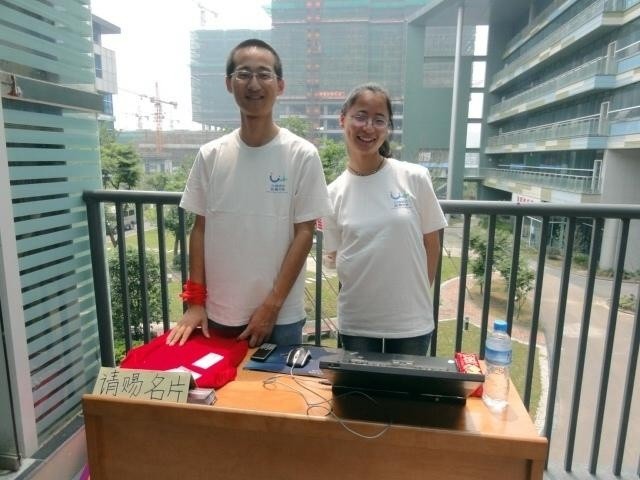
319,360,485,406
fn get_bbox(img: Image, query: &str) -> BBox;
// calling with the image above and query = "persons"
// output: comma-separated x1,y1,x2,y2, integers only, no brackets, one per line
322,85,450,356
165,39,336,348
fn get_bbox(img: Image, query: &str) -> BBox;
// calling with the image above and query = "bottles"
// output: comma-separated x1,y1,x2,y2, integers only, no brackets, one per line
482,320,512,409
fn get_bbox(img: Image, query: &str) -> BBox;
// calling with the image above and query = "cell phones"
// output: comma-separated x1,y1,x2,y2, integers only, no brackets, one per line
251,343,277,361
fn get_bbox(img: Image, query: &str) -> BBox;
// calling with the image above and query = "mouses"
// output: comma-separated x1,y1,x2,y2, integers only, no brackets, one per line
286,346,313,368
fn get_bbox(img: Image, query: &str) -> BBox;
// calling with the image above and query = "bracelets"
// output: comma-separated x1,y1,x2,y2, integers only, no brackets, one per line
180,281,207,306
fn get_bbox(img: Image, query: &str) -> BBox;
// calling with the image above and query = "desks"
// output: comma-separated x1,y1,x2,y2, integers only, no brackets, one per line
80,344,548,480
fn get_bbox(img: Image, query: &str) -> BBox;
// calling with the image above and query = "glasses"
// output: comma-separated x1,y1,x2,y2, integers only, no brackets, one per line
350,113,389,128
230,70,279,81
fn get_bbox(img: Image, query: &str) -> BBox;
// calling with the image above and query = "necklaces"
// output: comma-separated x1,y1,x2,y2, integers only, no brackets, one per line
349,155,385,177
